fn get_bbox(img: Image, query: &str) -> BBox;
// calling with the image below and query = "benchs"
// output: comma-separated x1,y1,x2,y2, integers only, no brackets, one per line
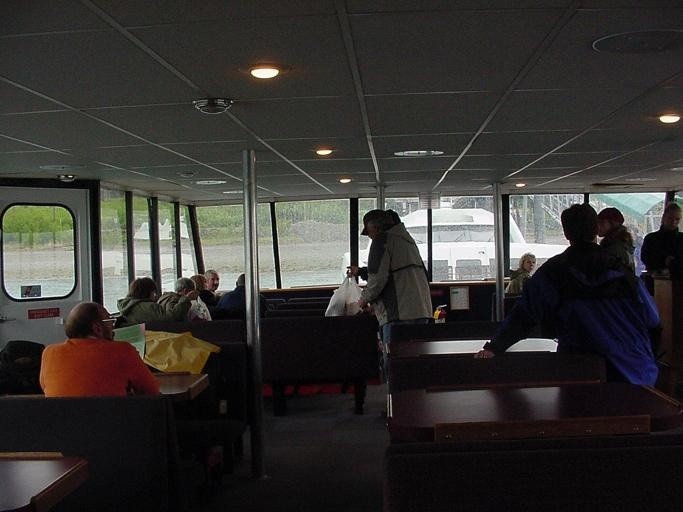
148,290,377,416
386,352,558,446
386,319,543,338
2,342,249,509
382,432,680,510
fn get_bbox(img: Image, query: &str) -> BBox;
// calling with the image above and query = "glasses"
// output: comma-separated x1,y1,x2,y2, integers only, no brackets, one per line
102,317,116,326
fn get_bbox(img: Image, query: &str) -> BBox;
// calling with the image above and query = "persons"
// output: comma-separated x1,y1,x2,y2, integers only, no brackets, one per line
40,301,160,397
505,255,534,292
347,209,433,419
204,270,221,305
215,274,268,321
119,278,177,330
596,207,635,276
191,274,216,321
640,202,683,279
477,203,660,388
157,277,199,320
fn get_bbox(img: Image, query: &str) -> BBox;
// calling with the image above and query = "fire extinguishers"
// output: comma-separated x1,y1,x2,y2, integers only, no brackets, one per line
433,305,448,325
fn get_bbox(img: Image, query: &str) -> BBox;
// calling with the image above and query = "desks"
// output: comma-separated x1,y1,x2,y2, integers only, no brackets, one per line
388,383,679,443
386,336,562,381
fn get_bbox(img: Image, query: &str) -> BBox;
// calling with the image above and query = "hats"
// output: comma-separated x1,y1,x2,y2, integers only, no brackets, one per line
360,210,385,235
598,208,624,223
